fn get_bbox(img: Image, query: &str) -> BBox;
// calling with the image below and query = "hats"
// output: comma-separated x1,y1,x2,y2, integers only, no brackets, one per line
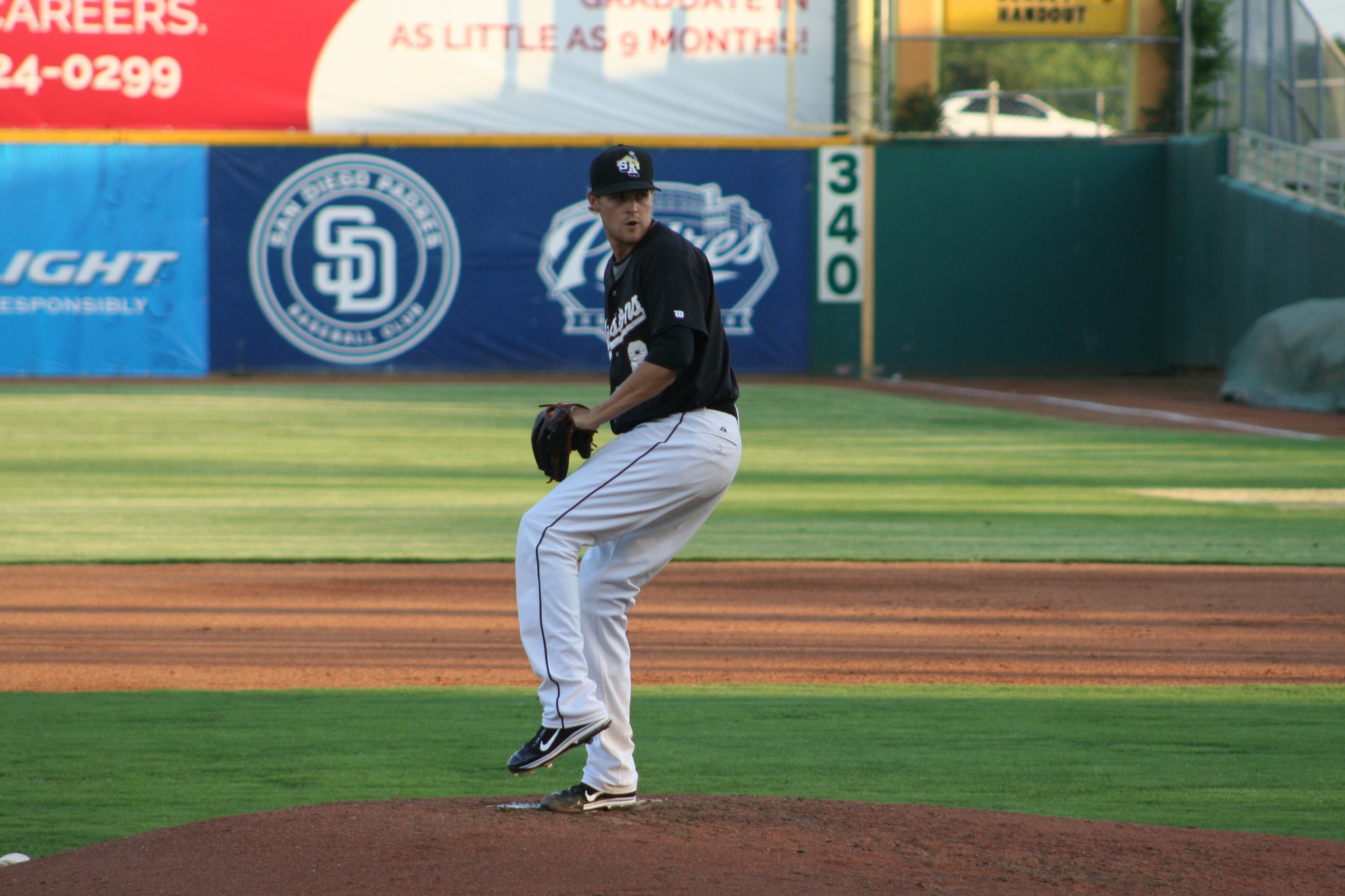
590,143,661,195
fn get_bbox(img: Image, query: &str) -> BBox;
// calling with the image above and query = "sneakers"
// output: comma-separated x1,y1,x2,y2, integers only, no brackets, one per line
507,718,612,776
541,783,637,814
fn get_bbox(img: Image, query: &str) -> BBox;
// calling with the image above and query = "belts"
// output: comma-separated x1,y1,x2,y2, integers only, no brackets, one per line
707,401,739,417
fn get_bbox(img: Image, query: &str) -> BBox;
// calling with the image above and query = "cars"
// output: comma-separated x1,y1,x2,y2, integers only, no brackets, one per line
936,89,1121,137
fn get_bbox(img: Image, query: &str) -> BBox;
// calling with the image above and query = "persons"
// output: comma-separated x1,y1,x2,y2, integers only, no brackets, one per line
505,143,742,815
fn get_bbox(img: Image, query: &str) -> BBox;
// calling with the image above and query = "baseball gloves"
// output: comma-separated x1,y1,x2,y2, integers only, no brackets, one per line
531,401,599,483
